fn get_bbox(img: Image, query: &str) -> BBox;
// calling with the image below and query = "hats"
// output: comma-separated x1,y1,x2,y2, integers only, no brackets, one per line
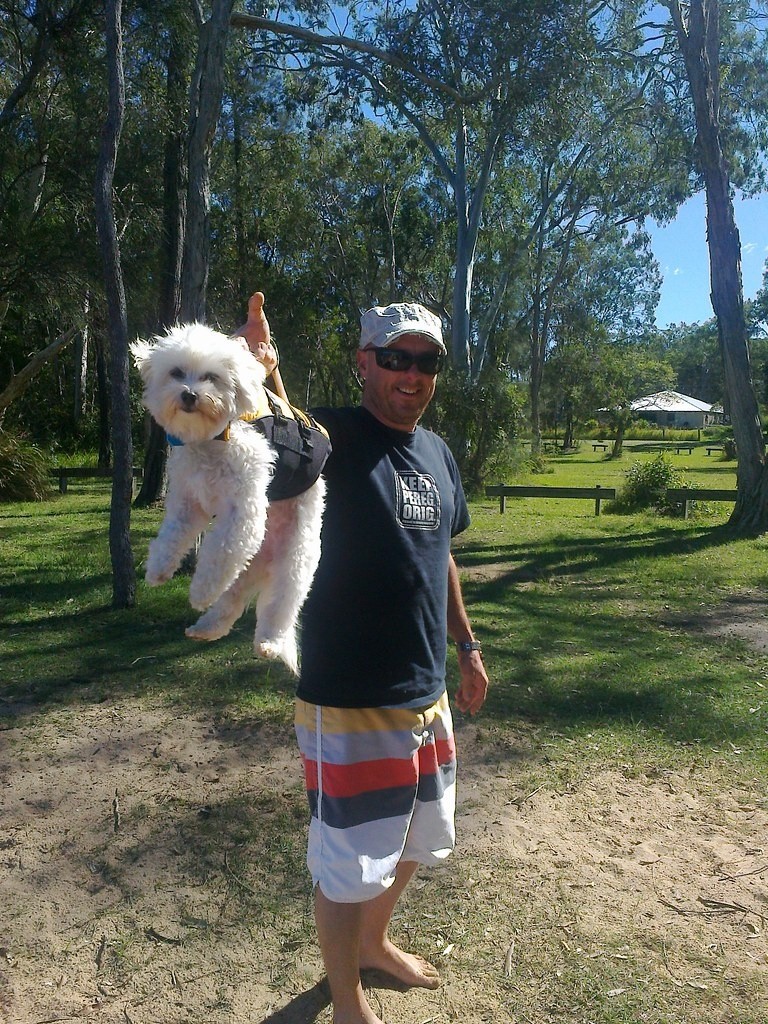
360,303,447,356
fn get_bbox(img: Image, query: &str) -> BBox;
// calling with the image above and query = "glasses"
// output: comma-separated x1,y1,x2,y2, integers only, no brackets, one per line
362,347,446,374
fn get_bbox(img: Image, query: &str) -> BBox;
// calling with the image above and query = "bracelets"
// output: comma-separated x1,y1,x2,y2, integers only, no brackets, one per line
454,641,481,652
270,340,280,373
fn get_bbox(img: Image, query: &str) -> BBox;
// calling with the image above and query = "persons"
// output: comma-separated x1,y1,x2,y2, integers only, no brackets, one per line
228,290,489,1024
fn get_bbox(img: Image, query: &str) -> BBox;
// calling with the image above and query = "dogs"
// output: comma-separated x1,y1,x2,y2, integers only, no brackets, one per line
127,320,328,679
